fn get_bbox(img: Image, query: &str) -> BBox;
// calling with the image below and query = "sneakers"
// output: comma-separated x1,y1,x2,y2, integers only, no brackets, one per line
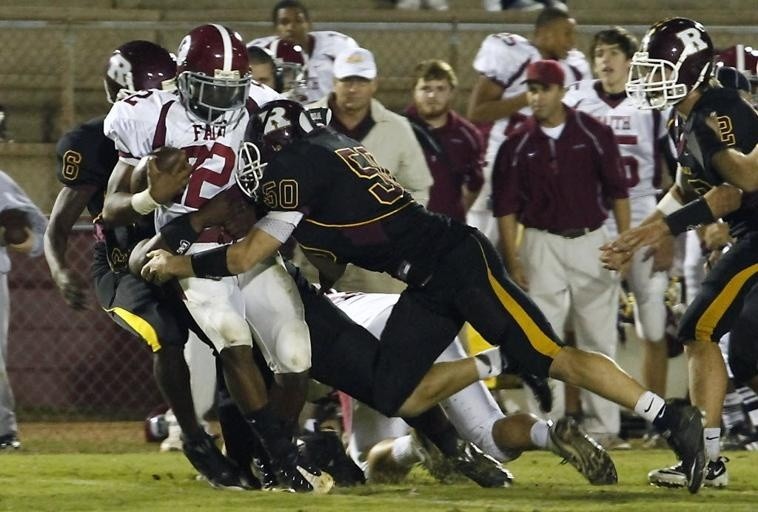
547,414,619,486
267,444,337,496
720,425,758,453
587,433,633,452
452,439,516,490
646,455,730,490
247,452,297,494
410,426,461,485
180,433,244,490
160,429,186,455
641,430,673,450
0,431,22,451
654,396,707,496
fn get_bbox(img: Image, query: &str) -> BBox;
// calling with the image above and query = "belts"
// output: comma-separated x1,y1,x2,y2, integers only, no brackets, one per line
518,218,607,238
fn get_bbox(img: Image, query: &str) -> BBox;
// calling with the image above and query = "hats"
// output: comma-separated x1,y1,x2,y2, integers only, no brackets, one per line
519,59,567,88
330,45,379,82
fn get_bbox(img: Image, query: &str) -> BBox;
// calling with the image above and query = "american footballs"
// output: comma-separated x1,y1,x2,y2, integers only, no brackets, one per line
129,148,179,192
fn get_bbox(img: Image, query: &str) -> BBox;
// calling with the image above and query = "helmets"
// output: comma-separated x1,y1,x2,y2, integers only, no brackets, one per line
233,99,319,201
101,40,180,105
246,36,309,93
624,15,716,113
713,42,758,107
175,23,253,129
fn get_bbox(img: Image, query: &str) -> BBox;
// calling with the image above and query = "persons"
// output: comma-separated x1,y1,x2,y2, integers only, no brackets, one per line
0,170,48,450
44,1,758,493
